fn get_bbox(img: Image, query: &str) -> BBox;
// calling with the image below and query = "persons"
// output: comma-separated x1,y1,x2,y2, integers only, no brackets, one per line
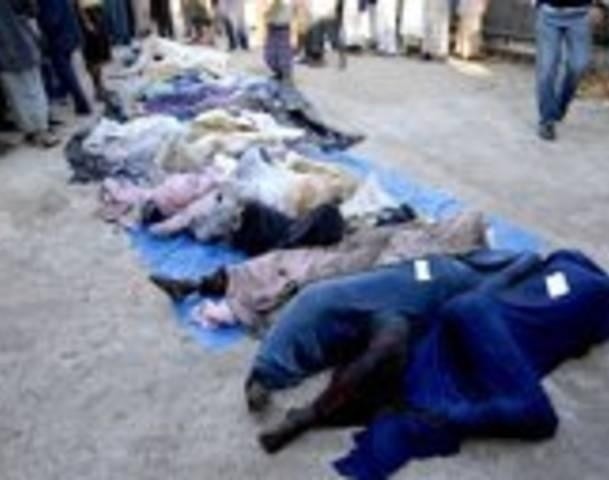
529,1,608,139
1,1,249,148
261,1,487,88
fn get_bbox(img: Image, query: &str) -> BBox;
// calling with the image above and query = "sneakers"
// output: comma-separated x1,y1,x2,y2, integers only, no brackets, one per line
537,116,557,140
